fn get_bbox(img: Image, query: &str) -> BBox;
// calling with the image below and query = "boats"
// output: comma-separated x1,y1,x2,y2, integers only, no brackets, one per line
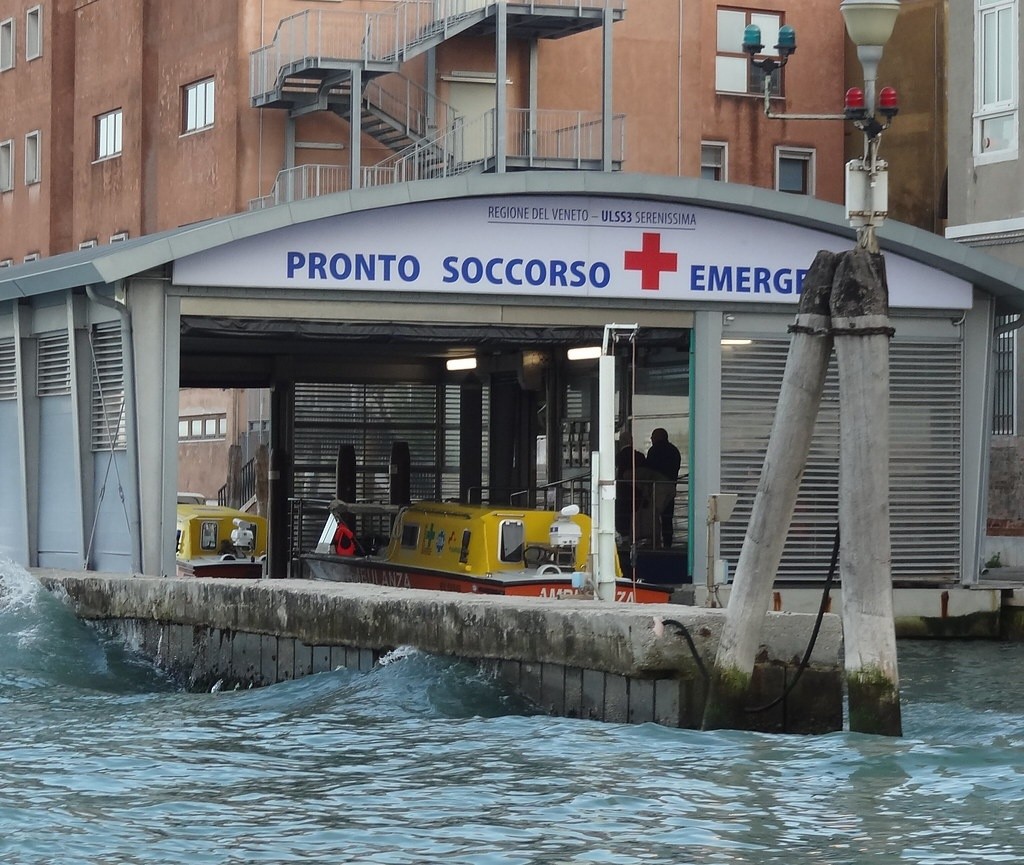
173,502,270,579
293,497,675,606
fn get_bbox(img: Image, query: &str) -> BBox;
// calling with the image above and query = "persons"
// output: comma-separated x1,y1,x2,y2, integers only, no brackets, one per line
615,428,681,553
